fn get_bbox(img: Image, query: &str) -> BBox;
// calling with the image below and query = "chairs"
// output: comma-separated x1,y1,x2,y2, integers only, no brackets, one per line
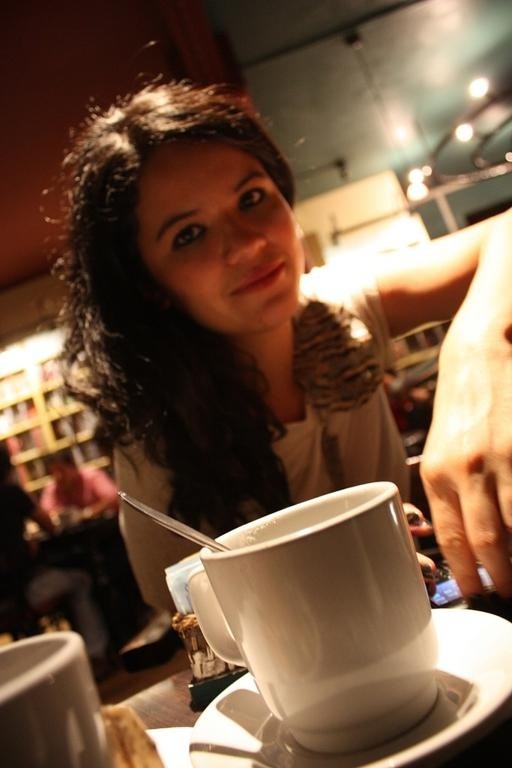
120,453,435,673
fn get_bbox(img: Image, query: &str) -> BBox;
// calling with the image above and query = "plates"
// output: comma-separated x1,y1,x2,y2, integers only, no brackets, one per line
186,606,512,767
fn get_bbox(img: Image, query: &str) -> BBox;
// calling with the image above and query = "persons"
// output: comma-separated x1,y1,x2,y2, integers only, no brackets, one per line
45,79,510,614
38,446,121,530
1,442,117,687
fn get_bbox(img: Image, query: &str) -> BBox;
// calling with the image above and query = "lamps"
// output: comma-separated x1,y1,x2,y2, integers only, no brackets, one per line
403,73,512,205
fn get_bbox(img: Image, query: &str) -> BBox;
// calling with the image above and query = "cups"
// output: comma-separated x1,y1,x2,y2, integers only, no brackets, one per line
0,629,114,768
186,480,442,756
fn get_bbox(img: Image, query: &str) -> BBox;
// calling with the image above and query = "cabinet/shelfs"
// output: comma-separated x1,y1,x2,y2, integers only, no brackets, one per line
389,319,450,369
0,353,114,534
